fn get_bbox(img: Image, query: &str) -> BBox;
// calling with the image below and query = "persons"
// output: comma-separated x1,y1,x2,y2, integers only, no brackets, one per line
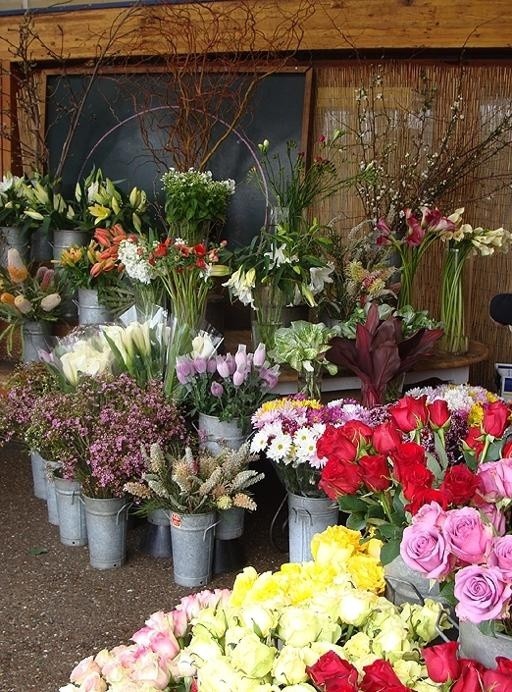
489,292,512,334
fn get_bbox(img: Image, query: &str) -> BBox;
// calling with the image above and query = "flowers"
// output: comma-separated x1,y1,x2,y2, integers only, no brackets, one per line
1,62,511,587
61,587,512,692
